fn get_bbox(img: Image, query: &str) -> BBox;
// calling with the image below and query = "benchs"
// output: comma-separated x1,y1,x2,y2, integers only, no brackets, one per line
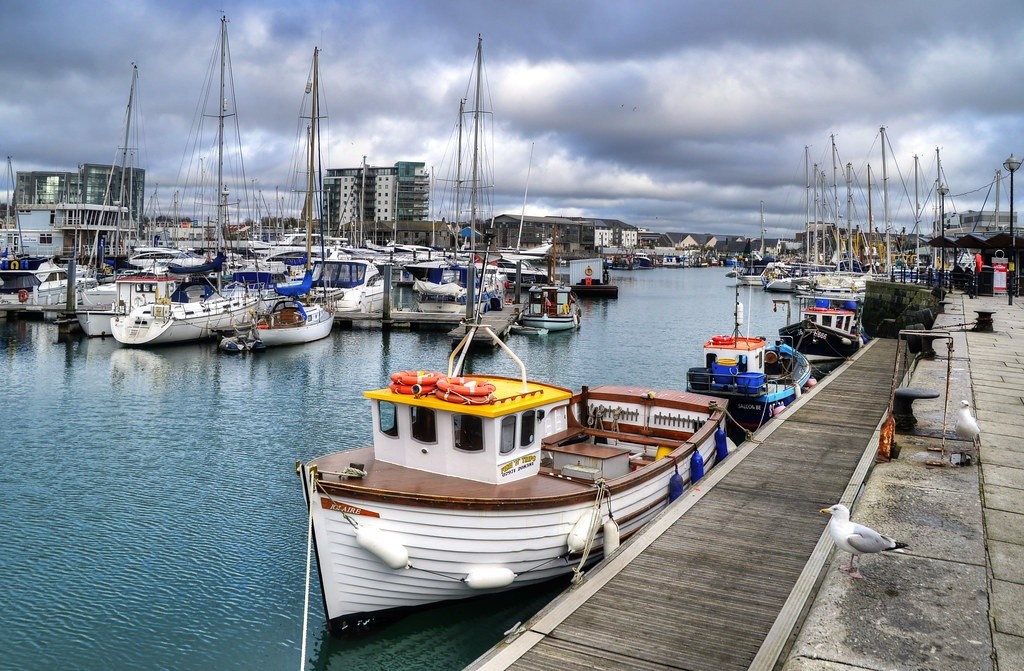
542,404,585,445
581,418,695,448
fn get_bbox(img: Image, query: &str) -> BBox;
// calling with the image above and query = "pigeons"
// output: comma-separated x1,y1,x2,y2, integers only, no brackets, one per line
819,503,913,579
955,400,981,450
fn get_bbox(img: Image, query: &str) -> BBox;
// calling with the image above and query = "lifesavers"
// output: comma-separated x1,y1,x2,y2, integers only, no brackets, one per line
387,368,496,406
584,267,593,276
11,261,19,270
709,335,735,345
17,290,29,302
563,303,569,314
809,306,827,311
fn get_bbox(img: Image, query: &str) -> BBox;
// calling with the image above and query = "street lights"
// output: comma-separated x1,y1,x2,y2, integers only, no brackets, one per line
1003,153,1022,305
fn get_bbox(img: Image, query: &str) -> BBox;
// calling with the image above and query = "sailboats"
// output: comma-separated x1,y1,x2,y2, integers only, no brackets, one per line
725,125,976,301
0,9,553,351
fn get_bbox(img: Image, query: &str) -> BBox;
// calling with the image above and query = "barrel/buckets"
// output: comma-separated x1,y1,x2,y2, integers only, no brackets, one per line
843,301,857,310
735,372,764,393
974,266,994,296
712,359,739,384
816,299,829,308
490,296,503,311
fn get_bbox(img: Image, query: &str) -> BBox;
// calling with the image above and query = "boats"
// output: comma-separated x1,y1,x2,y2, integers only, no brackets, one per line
516,220,582,332
684,300,812,446
774,294,864,362
295,213,737,631
